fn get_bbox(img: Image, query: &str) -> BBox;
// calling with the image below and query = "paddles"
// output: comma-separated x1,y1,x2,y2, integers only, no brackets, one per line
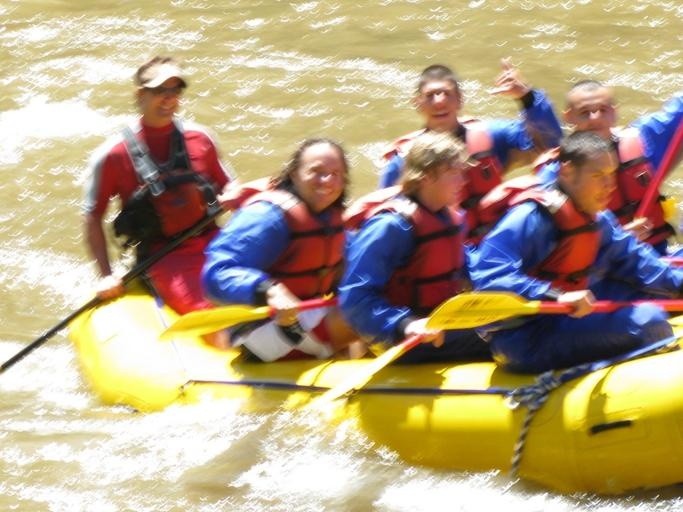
300,333,430,412
161,296,338,342
425,290,682,333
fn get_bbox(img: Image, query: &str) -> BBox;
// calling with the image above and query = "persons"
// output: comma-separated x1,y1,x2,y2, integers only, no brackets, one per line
381,54,562,211
196,134,370,364
523,67,683,271
463,129,683,377
77,53,259,351
332,129,492,362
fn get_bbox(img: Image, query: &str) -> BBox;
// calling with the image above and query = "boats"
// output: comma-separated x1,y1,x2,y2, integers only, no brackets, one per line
71,252,682,500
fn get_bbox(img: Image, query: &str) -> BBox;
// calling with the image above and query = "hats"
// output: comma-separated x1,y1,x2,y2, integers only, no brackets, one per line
137,64,187,90
